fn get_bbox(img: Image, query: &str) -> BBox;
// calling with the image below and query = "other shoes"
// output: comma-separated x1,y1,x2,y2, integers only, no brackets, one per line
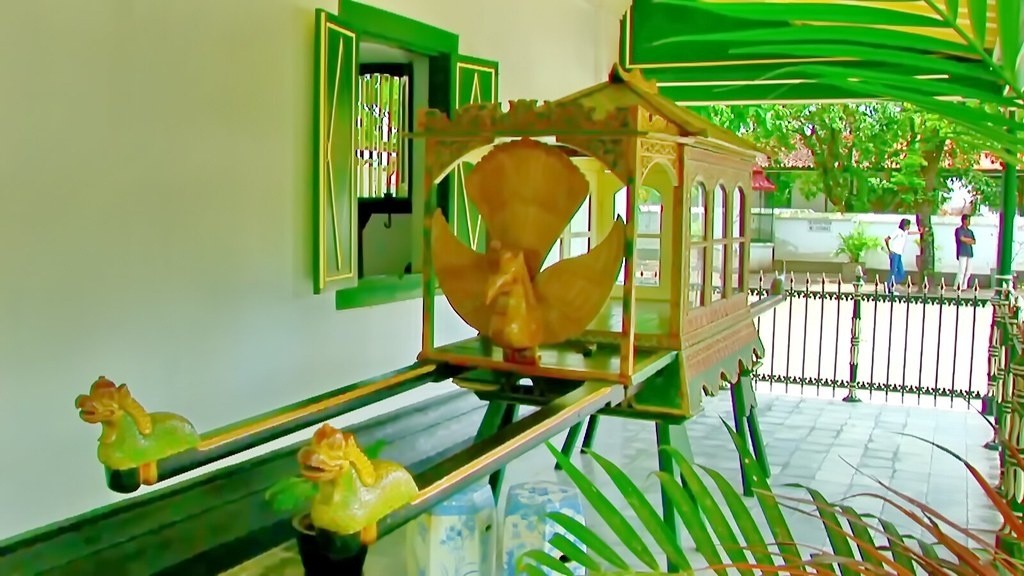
893,292,899,295
884,281,889,294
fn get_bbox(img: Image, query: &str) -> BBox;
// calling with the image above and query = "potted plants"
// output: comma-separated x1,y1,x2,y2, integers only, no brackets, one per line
833,219,883,283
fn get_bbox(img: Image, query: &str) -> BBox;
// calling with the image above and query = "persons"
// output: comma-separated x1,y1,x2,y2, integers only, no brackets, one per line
954,214,976,292
884,219,923,296
